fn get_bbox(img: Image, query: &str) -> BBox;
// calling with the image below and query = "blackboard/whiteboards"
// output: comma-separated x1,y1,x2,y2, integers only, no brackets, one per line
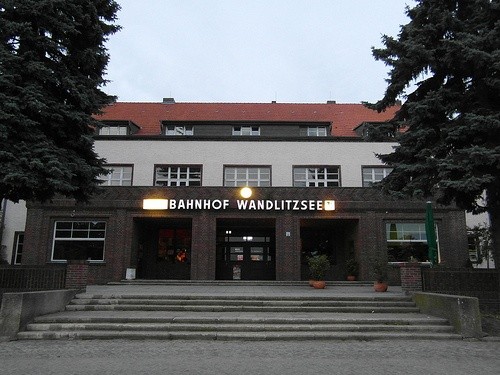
233,264,241,280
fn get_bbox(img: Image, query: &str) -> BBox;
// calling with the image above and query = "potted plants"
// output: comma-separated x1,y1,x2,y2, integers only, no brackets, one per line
306,255,330,288
344,258,358,281
367,252,390,292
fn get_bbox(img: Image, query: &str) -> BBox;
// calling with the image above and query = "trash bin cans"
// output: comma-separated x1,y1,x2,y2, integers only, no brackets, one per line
125,267,136,281
